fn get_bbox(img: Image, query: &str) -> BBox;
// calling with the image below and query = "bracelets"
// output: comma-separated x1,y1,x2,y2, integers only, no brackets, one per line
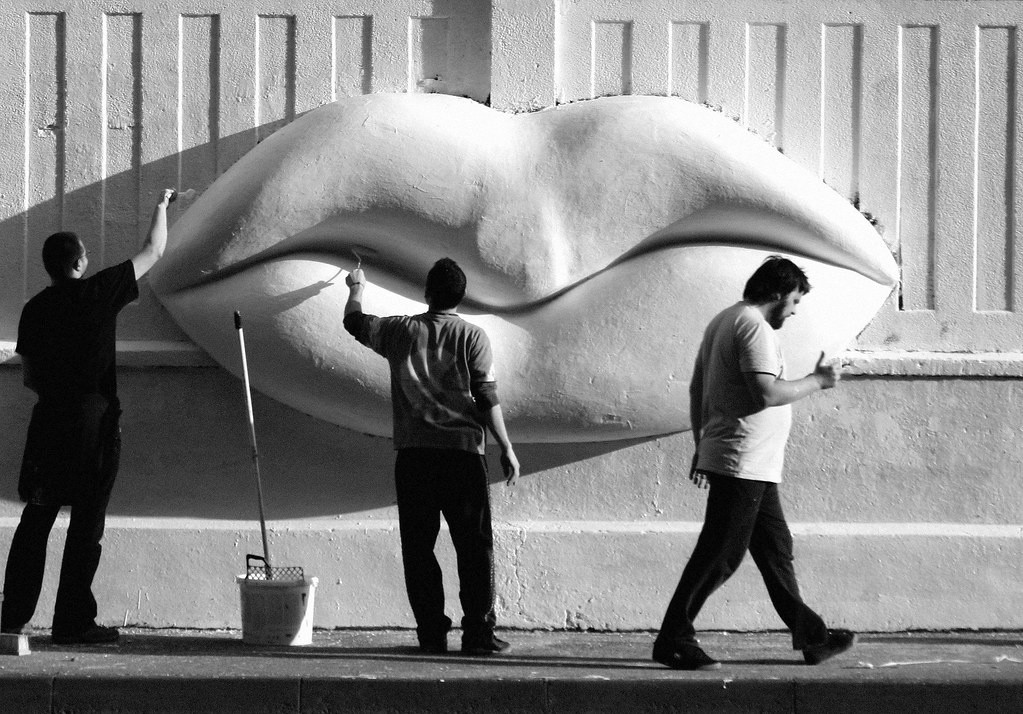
350,282,365,288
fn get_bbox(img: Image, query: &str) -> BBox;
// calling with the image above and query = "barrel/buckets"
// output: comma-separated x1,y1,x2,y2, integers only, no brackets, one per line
235,573,319,645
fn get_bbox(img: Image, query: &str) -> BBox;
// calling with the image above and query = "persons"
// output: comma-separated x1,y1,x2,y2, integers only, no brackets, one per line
649,250,851,672
342,257,522,656
0,188,177,646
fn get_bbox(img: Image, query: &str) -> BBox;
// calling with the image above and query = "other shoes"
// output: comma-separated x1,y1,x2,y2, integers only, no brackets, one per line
419,638,446,654
652,640,721,672
461,637,513,657
803,629,855,666
51,623,118,648
